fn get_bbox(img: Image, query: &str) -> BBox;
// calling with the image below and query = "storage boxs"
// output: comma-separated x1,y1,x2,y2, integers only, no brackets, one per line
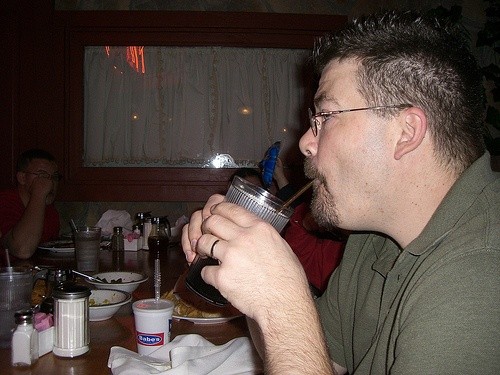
37,326,54,357
124,235,143,251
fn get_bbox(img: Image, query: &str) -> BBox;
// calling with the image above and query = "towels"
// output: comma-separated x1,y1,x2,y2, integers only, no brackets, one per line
106,334,264,375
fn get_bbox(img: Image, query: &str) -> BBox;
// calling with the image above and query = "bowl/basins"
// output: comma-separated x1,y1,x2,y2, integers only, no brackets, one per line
87,288,133,321
84,269,150,295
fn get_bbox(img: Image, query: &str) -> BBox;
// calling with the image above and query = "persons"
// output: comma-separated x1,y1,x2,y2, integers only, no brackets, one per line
182,7,500,375
0,148,65,260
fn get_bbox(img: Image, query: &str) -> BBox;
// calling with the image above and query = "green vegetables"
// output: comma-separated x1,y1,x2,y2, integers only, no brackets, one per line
91,276,121,284
88,299,109,306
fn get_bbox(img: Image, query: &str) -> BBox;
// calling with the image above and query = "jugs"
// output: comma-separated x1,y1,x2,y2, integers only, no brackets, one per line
148,214,171,256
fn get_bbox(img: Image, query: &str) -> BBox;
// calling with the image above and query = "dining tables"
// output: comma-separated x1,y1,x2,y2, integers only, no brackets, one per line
0,235,267,375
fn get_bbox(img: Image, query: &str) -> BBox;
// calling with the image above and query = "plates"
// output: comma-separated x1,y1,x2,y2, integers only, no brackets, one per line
172,312,243,325
259,141,280,190
38,241,75,252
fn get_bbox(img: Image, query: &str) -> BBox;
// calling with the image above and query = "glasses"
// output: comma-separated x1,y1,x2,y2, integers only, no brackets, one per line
304,104,414,138
22,169,62,183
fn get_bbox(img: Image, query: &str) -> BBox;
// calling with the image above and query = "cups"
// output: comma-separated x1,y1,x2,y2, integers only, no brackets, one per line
0,266,36,351
73,224,102,275
175,173,299,313
132,298,175,355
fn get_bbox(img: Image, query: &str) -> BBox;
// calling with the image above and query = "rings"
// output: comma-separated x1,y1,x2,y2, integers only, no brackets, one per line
210,239,219,256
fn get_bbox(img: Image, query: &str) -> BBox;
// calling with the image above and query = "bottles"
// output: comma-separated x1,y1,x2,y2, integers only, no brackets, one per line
133,211,152,251
111,226,124,254
9,310,40,368
52,284,91,360
46,266,72,304
